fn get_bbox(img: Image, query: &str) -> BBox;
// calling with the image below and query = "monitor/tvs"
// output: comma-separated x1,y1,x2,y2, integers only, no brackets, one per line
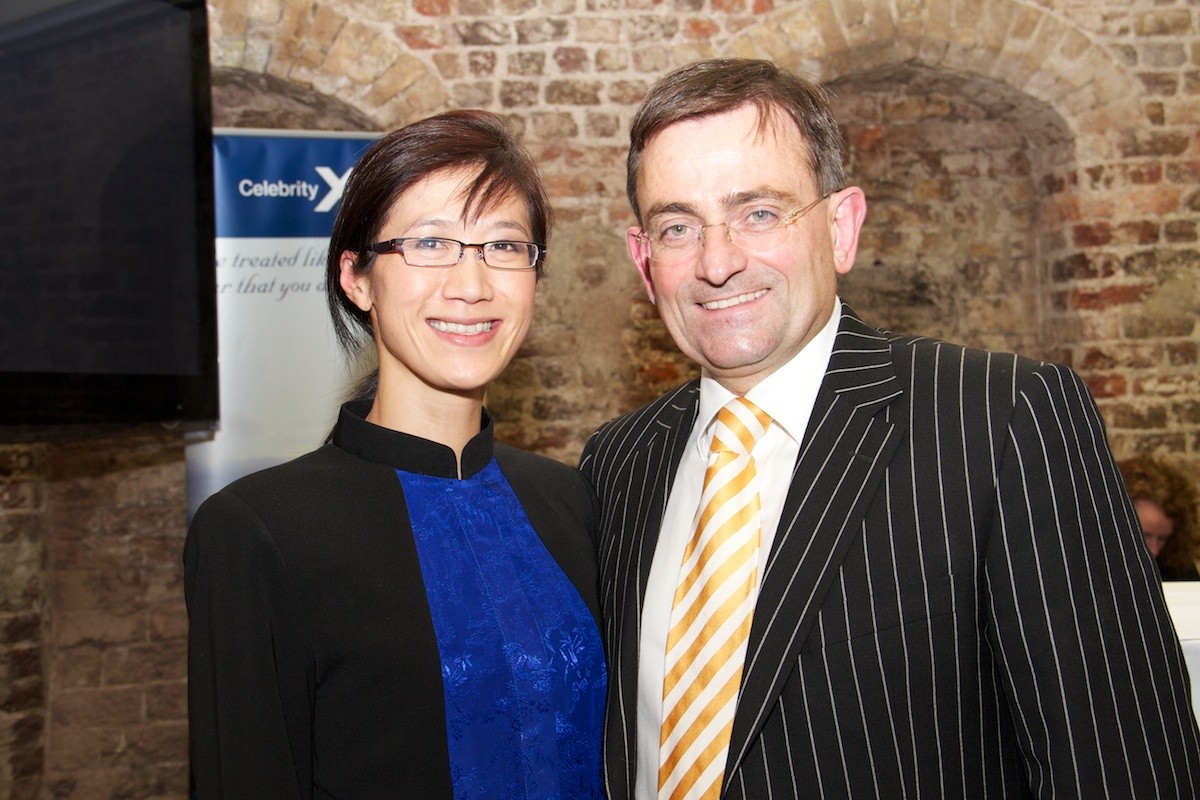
0,1,218,427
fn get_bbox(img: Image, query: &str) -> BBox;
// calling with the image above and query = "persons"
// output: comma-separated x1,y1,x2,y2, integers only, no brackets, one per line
184,110,609,800
578,57,1200,800
1122,456,1200,581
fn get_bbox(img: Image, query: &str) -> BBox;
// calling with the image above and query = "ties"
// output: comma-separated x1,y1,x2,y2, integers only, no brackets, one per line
657,396,771,800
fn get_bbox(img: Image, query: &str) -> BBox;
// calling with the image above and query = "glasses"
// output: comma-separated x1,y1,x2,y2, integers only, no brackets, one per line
633,187,843,267
363,237,547,270
